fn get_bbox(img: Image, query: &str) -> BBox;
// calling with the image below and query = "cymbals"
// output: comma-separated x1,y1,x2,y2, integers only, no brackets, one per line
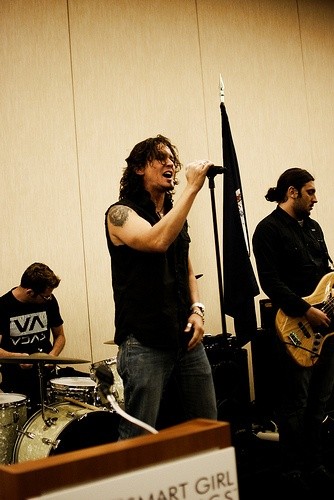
0,353,91,364
103,340,116,345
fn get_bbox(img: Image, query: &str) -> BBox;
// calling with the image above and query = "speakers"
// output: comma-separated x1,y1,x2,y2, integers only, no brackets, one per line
209,350,251,434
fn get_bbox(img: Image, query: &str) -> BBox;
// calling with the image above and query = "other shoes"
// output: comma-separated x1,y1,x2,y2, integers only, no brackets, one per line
287,465,303,482
312,465,332,480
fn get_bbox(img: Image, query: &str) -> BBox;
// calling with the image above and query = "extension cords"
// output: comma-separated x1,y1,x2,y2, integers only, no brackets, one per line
256,431,279,441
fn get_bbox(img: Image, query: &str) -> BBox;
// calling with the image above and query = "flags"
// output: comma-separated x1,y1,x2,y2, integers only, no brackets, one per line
220,102,260,351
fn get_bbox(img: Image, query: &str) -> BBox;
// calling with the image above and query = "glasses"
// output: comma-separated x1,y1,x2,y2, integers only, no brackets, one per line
26,288,51,302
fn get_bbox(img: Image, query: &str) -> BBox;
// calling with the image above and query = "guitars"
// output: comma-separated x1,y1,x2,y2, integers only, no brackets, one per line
275,272,334,367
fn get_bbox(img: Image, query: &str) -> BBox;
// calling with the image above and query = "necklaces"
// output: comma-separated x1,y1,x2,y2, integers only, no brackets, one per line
156,202,164,217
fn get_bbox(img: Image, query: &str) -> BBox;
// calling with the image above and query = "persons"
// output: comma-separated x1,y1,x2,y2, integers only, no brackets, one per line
251,167,334,495
103,134,215,441
0,262,91,419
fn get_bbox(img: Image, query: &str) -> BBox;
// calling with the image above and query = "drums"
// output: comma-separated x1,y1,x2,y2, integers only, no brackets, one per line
46,376,98,405
89,356,125,410
0,393,29,464
13,401,120,465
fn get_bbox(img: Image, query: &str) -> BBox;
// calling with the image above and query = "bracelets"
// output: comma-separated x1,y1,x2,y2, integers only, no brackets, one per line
189,303,205,325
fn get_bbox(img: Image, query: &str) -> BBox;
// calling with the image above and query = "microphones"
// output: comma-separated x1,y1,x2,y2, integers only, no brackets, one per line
205,165,226,176
